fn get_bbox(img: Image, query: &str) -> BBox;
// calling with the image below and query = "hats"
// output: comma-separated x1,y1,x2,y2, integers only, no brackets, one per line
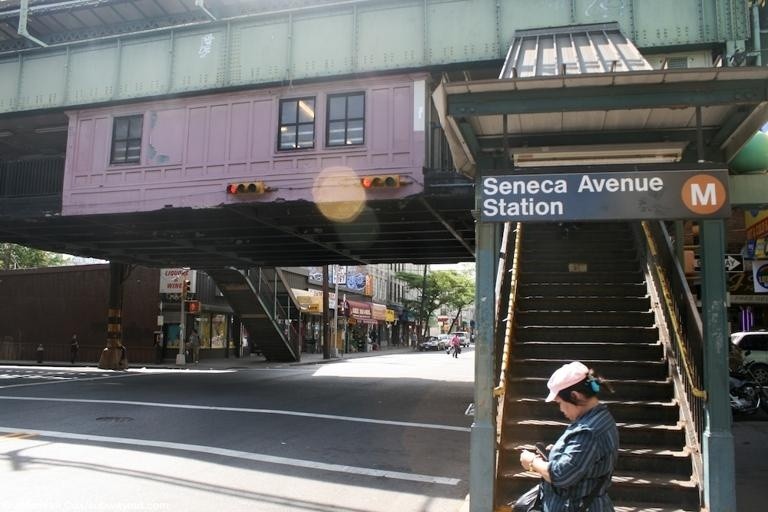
545,361,589,403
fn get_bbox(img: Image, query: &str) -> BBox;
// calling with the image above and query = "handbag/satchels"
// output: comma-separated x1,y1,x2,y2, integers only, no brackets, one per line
512,483,542,512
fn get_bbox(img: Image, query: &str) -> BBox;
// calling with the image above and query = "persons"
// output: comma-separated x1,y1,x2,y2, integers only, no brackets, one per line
450,333,461,359
69,332,80,364
518,361,620,512
189,328,201,365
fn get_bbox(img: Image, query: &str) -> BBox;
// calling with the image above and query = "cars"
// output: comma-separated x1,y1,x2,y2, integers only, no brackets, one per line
418,334,451,351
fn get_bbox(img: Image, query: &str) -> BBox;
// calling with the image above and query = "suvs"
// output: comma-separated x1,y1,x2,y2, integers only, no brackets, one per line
731,331,768,386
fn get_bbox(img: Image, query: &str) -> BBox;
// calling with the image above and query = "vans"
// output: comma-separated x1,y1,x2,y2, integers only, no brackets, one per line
452,331,470,348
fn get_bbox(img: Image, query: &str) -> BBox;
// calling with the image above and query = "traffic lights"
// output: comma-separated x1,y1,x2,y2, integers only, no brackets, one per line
226,180,265,197
359,175,401,189
183,279,191,298
188,300,201,315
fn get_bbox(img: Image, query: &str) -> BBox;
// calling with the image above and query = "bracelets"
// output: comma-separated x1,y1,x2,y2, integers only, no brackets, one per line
529,453,539,474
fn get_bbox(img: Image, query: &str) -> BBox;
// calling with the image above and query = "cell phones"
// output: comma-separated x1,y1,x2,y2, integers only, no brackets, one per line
535,442,549,461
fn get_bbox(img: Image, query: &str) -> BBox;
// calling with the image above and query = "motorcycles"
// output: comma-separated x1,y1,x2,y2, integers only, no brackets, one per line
701,350,768,418
